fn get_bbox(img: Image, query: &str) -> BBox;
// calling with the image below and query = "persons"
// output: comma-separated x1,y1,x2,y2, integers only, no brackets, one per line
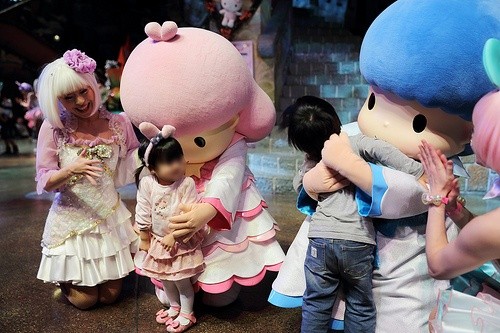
418,88,500,333
34,57,142,310
133,135,206,333
0,82,43,158
281,95,429,333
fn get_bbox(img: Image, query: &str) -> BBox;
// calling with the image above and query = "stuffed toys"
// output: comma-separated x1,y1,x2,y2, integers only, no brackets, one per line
120,21,285,308
268,0,500,333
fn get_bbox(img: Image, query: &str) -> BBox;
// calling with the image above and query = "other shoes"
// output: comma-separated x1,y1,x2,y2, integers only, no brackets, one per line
156,306,196,333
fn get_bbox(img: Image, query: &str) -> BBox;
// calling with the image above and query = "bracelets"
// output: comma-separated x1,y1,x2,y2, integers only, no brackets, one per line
422,192,448,206
446,195,466,217
